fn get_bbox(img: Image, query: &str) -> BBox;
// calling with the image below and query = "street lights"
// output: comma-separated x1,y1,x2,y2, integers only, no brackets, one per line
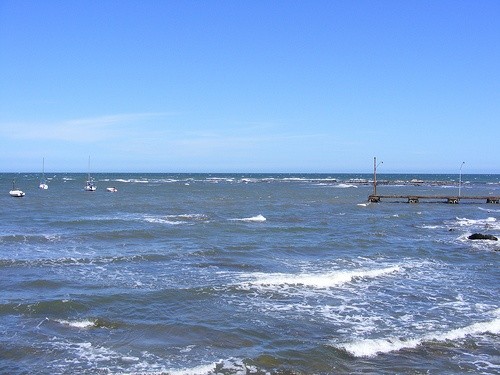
458,161,465,196
373,156,384,195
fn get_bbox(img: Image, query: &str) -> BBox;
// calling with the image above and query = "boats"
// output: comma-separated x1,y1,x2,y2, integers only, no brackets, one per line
105,188,118,192
9,190,26,197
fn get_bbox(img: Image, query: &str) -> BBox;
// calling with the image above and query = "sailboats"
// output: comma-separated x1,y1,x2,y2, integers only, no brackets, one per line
84,153,97,191
39,156,49,189
